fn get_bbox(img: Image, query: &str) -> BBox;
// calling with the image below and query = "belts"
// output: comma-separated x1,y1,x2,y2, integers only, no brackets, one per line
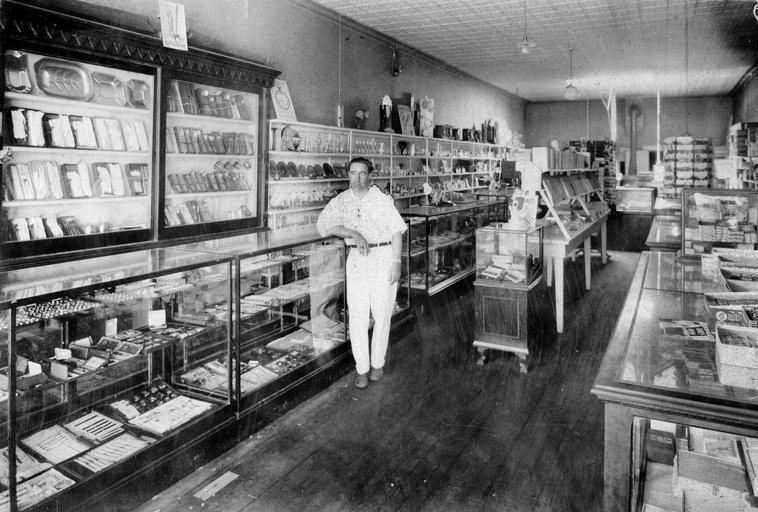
349,241,391,248
321,242,330,245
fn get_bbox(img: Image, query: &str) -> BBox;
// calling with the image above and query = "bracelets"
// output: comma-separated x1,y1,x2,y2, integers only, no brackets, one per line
387,256,401,264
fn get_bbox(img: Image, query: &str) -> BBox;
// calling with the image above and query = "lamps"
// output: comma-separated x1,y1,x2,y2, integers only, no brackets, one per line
563,45,578,100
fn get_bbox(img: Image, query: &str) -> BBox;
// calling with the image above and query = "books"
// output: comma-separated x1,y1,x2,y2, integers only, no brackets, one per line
482,248,526,284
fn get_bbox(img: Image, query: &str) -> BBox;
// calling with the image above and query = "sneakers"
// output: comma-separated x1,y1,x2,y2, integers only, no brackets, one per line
369,366,384,382
354,373,369,389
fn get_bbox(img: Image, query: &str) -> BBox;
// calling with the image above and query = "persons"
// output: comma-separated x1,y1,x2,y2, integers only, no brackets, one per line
316,156,410,391
